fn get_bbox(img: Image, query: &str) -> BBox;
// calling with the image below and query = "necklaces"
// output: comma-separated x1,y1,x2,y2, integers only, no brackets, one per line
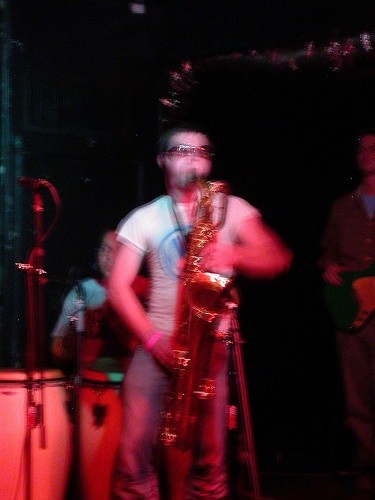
169,197,188,249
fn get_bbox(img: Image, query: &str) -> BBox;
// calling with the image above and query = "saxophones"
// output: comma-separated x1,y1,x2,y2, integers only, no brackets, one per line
151,168,234,455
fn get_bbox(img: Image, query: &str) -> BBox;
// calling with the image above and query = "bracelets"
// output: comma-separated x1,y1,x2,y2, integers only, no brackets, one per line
143,330,164,355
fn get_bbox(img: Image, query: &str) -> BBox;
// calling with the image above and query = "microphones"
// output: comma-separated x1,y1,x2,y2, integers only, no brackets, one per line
76,281,86,312
17,176,46,190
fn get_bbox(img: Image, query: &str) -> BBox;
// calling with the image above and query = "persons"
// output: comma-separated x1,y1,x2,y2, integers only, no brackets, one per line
106,115,290,500
314,130,375,494
50,226,155,500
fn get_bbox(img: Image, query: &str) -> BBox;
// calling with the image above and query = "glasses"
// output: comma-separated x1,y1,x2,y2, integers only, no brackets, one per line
162,145,213,160
